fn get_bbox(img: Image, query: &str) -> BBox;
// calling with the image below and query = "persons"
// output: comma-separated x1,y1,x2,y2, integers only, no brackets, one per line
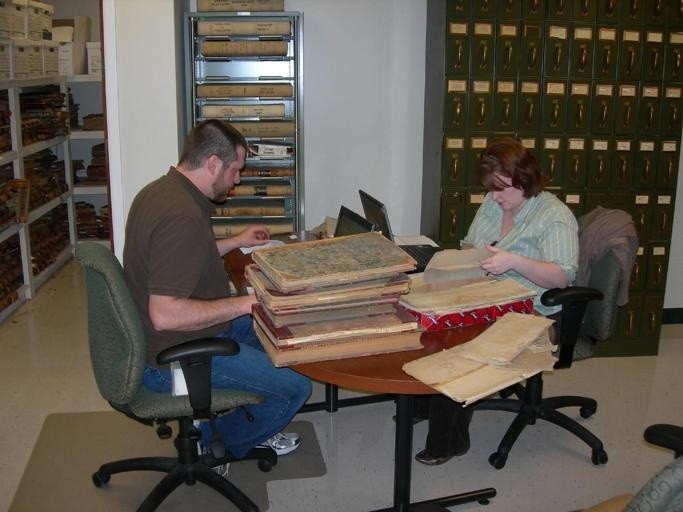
122,118,313,476
392,137,581,466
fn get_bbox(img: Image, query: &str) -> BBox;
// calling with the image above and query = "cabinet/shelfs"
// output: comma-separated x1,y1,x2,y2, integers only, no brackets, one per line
184,10,306,236
439,0,683,359
0,73,112,324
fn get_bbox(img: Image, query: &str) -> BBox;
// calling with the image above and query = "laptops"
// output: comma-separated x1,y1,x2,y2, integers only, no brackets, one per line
359,189,437,275
334,205,375,237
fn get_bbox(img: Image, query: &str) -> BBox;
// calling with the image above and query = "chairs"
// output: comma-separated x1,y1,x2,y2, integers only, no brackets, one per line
449,205,639,469
75,241,278,511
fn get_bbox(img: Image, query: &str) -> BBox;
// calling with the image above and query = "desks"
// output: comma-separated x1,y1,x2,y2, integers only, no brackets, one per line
222,228,557,511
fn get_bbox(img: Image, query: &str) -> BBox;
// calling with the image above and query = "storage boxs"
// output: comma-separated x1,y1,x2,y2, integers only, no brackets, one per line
0,0,103,81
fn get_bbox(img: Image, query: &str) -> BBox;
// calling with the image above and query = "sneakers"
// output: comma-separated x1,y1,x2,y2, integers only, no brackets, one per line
255,431,301,457
415,449,450,465
193,419,231,478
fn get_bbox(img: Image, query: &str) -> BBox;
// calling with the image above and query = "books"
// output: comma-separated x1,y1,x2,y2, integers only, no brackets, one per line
398,248,537,315
244,232,427,367
458,311,557,366
